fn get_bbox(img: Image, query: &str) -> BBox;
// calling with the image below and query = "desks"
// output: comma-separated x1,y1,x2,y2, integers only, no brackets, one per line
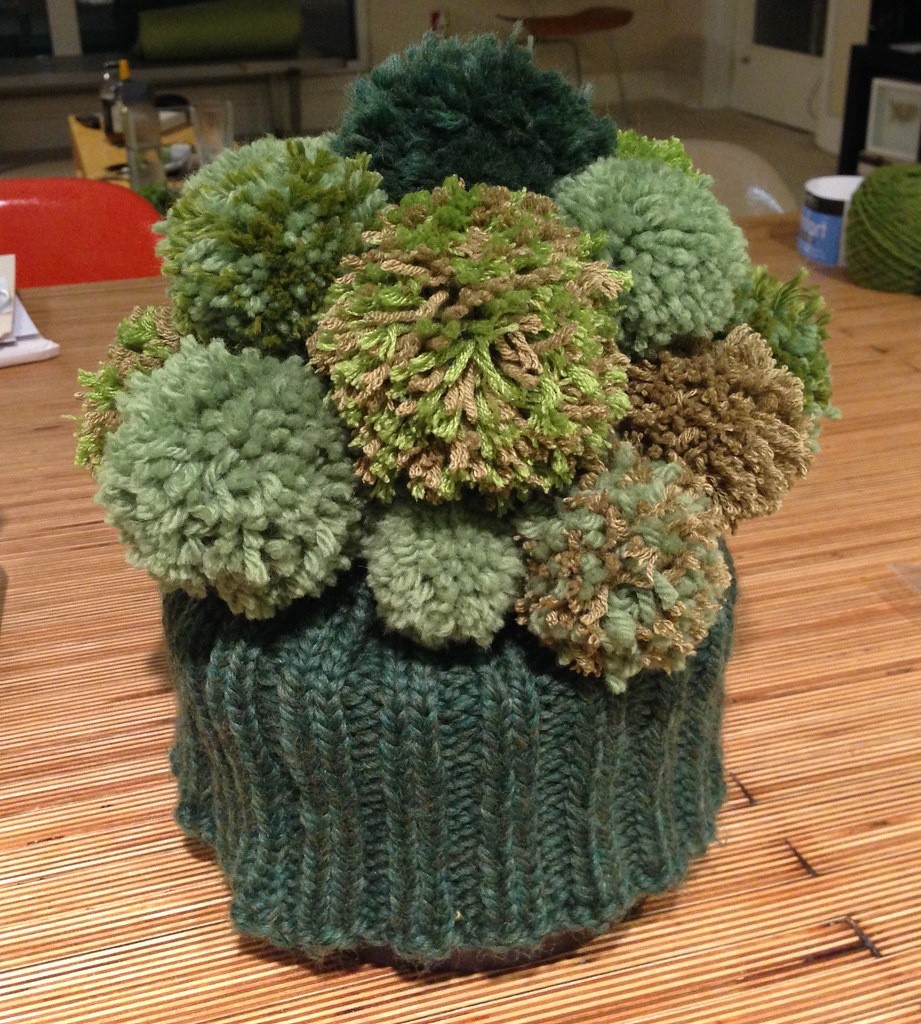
0,211,920,1021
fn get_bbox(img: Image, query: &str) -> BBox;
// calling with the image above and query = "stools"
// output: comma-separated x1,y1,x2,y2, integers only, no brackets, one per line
493,5,636,121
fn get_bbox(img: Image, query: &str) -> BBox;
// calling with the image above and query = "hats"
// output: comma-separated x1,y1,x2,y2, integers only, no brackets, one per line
66,31,842,982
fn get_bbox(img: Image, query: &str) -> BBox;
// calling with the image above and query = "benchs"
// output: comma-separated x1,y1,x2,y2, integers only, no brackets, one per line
0,47,304,138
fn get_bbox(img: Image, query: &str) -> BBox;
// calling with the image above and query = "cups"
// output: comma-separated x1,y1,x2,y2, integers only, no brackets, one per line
190,100,235,161
120,104,165,189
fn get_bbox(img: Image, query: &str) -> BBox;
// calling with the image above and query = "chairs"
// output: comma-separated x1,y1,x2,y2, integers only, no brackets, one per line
672,139,802,240
0,177,187,290
